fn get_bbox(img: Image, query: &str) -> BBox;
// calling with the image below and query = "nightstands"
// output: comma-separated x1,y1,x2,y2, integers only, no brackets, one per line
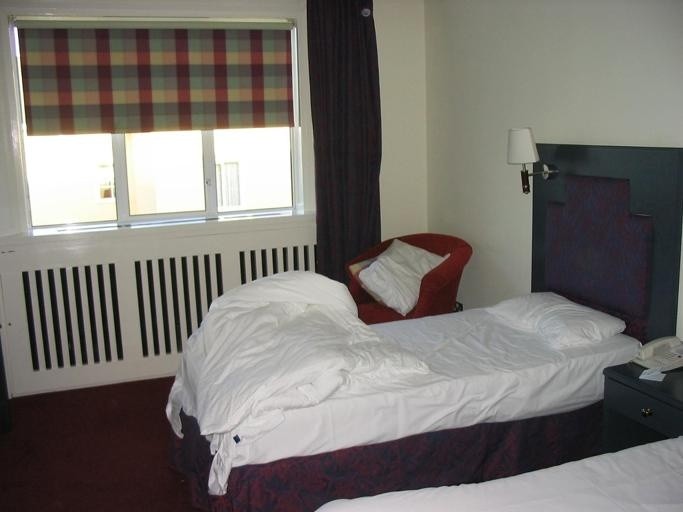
601,360,683,452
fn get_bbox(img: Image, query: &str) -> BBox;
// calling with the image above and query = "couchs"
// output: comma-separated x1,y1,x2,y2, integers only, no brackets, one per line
343,233,473,325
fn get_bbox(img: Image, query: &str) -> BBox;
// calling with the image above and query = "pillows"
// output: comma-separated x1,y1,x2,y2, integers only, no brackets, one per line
348,256,386,309
358,239,449,316
488,291,626,350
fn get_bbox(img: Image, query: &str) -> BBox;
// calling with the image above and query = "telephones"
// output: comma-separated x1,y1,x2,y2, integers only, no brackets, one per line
632,335,683,373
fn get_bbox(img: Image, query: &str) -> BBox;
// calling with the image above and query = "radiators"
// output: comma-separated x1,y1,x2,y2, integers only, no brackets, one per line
1,226,316,400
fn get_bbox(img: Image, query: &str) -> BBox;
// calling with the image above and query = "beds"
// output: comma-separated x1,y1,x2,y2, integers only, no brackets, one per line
167,307,642,512
315,436,683,512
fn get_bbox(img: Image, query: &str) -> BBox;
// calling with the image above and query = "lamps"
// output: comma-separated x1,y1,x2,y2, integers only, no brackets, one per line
507,128,559,194
